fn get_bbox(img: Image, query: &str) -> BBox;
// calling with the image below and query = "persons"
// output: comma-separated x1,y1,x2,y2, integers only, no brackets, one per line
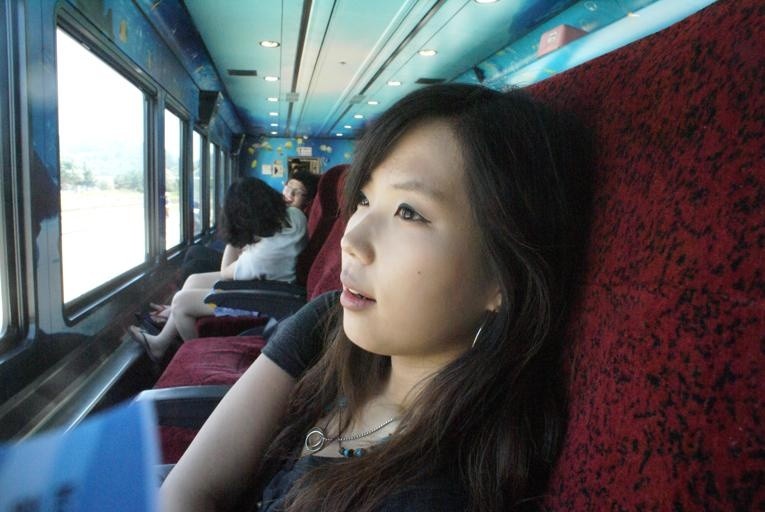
154,83,592,510
127,171,322,364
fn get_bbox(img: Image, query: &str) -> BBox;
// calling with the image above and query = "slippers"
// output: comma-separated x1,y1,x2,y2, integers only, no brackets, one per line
128,302,168,364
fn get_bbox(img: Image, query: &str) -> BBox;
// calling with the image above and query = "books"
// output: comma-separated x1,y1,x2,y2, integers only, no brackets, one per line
0,394,161,512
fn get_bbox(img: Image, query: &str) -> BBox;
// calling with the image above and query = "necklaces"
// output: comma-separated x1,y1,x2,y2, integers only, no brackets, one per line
304,396,403,459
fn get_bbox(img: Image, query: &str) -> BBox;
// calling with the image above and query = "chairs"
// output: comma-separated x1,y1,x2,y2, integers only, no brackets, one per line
141,1,763,512
